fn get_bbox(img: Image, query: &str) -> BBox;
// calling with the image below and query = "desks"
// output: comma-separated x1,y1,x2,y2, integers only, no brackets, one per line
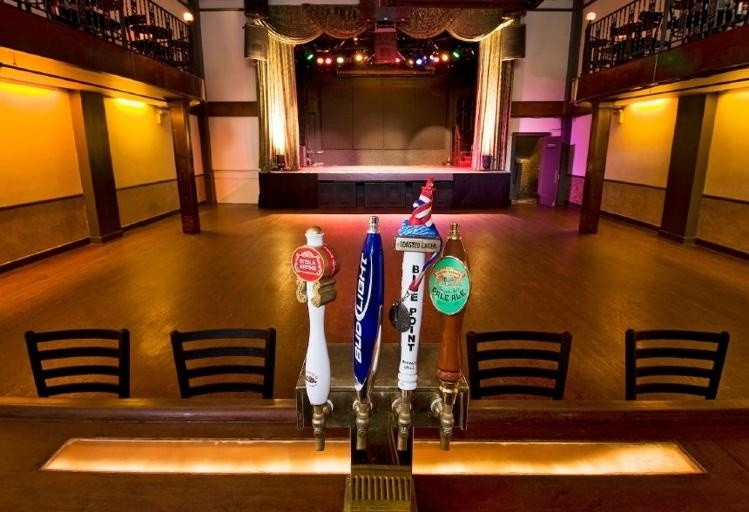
1,396,749,512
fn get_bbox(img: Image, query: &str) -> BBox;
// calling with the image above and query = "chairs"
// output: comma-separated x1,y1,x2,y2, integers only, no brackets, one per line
590,0,749,75
170,327,276,399
25,328,131,398
467,330,572,400
625,328,730,402
15,0,192,70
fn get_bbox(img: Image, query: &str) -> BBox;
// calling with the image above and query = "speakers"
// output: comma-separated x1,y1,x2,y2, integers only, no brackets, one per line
538,137,570,207
501,24,526,61
373,32,397,64
244,24,268,60
